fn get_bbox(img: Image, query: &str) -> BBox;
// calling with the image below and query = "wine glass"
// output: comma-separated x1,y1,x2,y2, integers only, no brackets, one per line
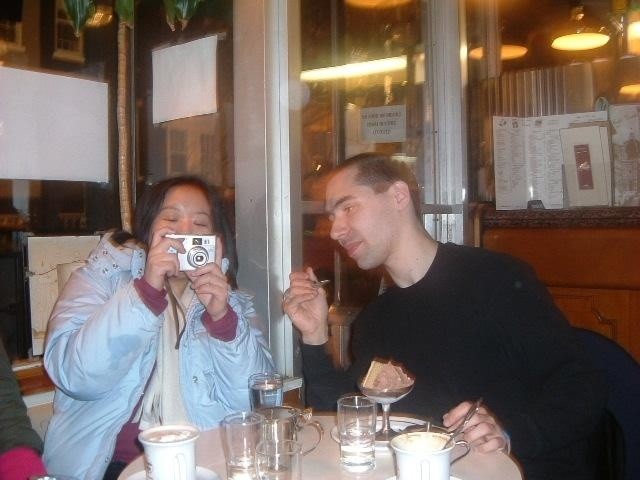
357,370,416,441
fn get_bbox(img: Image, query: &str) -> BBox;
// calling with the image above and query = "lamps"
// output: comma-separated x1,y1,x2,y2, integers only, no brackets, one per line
551,4,612,51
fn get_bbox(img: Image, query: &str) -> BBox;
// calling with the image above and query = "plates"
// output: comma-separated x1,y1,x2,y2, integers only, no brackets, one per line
123,464,219,480
332,417,428,449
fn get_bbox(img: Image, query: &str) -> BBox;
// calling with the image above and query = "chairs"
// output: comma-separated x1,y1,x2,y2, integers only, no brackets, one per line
575,326,640,480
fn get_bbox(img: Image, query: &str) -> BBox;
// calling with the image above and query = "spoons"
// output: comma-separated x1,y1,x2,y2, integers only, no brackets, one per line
309,277,330,289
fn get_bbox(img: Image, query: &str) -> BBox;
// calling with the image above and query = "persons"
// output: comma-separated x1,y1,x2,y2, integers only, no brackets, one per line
282,154,626,480
42,174,283,480
0,339,49,480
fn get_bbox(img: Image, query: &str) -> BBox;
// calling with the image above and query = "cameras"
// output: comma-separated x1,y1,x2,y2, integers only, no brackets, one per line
165,235,216,273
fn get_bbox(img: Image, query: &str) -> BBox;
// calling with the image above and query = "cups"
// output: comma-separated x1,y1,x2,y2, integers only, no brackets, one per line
253,438,305,480
336,396,377,474
221,410,265,480
390,431,472,477
259,404,322,458
135,424,199,480
247,371,283,409
